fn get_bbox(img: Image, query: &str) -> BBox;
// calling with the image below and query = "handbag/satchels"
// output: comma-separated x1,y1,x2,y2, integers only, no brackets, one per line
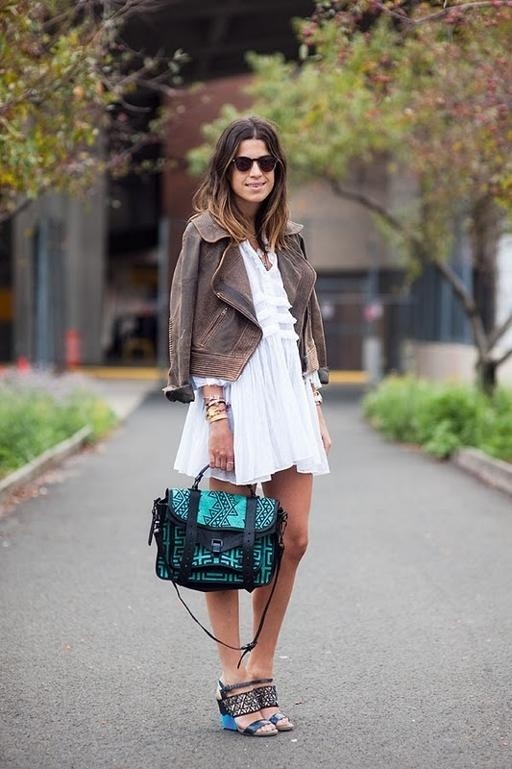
147,457,289,593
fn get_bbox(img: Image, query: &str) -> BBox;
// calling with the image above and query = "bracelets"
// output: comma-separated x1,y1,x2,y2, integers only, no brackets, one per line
204,391,232,424
314,390,322,405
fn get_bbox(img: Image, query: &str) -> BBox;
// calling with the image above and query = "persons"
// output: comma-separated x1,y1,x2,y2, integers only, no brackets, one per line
149,116,330,737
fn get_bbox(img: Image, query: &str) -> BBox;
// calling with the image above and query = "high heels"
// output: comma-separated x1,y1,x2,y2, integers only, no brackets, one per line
215,677,279,737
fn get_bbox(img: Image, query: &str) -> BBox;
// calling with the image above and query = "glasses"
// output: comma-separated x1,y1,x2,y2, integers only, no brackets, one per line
226,152,276,173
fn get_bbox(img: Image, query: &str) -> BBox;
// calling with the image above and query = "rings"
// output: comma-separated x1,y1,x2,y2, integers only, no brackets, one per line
227,462,233,464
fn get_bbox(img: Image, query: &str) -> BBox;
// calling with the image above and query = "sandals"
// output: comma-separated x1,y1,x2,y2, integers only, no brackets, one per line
244,669,294,732
